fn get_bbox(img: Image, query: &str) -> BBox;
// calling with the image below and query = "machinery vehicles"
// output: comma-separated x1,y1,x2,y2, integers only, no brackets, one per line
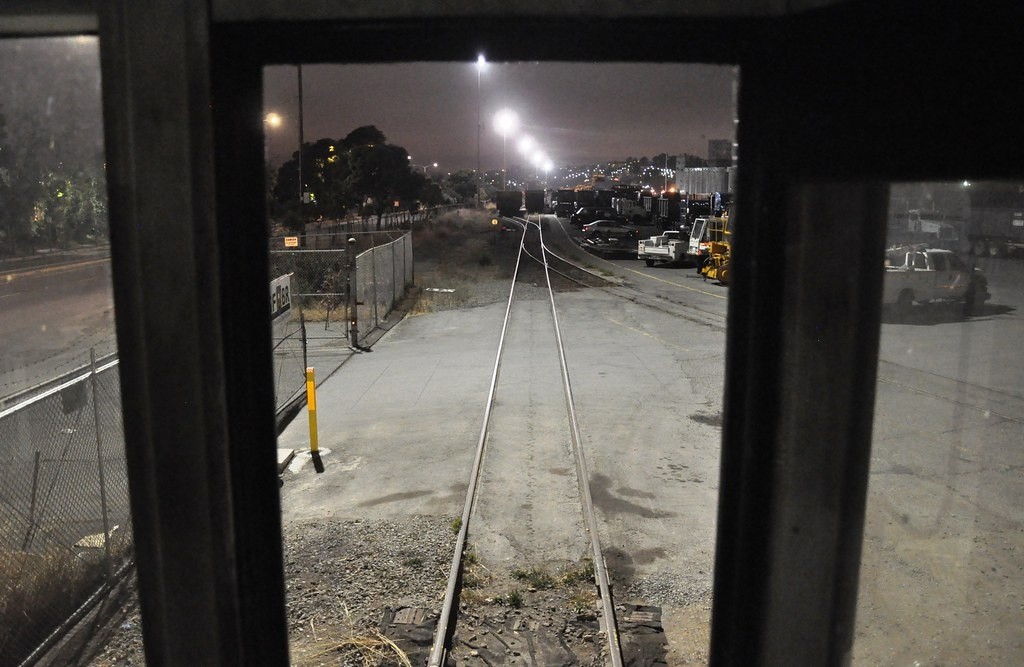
695,212,733,285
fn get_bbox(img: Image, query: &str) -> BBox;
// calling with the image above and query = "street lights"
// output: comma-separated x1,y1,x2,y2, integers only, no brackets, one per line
543,162,551,181
533,154,543,180
475,53,486,203
521,140,531,190
500,115,516,190
407,163,438,173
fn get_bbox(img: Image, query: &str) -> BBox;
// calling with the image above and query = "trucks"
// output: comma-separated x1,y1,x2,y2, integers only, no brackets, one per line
880,247,993,311
890,196,961,253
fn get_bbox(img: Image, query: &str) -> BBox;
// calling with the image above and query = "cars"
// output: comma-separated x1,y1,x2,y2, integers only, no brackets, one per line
550,196,725,268
